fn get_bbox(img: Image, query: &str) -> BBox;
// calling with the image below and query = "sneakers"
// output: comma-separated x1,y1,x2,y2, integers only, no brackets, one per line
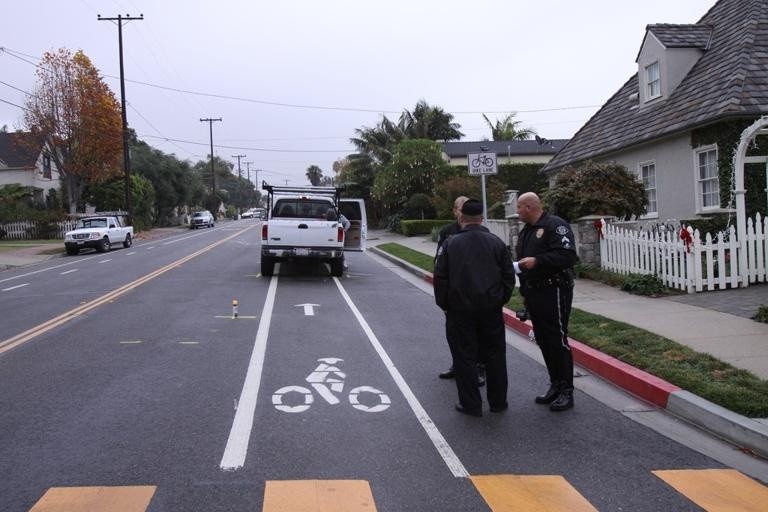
549,392,573,411
490,401,508,412
535,385,559,404
476,368,485,385
455,403,482,415
440,368,454,379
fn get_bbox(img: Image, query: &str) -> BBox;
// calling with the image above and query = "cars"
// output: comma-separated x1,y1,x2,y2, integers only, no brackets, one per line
189,209,215,230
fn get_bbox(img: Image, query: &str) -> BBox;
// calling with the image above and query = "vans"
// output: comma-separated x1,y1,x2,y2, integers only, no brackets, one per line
241,207,264,218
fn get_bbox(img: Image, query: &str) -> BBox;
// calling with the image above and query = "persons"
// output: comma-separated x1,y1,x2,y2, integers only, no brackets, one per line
515,191,577,411
434,196,490,386
433,199,515,416
336,207,350,232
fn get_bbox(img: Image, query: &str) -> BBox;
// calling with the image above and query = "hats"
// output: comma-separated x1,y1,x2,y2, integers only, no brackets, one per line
462,199,482,216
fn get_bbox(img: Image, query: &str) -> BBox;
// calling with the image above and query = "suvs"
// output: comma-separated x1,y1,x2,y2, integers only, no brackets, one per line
260,179,367,279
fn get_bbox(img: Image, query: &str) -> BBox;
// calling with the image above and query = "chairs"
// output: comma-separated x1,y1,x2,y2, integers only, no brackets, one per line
316,206,326,215
280,205,295,215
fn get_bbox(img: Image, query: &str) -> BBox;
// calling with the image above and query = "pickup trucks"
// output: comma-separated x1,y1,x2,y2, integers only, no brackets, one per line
65,216,135,253
